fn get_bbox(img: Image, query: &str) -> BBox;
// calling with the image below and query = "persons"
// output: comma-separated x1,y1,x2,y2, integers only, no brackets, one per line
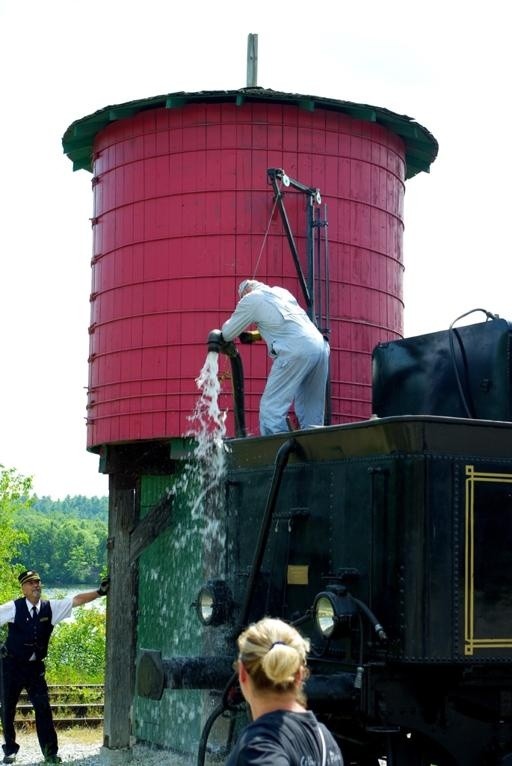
221,278,331,437
0,567,110,764
222,614,344,765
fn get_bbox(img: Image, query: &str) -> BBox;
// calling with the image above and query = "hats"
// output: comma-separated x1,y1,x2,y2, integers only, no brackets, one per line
18,570,41,586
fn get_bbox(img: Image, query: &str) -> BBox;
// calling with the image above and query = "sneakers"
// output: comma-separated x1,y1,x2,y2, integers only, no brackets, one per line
45,755,61,763
3,752,16,763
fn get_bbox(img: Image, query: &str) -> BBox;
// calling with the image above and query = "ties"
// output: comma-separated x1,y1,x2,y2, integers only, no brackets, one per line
32,606,38,625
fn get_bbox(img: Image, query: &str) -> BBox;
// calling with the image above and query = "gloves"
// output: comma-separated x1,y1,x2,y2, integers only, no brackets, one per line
0,642,9,659
96,576,110,596
